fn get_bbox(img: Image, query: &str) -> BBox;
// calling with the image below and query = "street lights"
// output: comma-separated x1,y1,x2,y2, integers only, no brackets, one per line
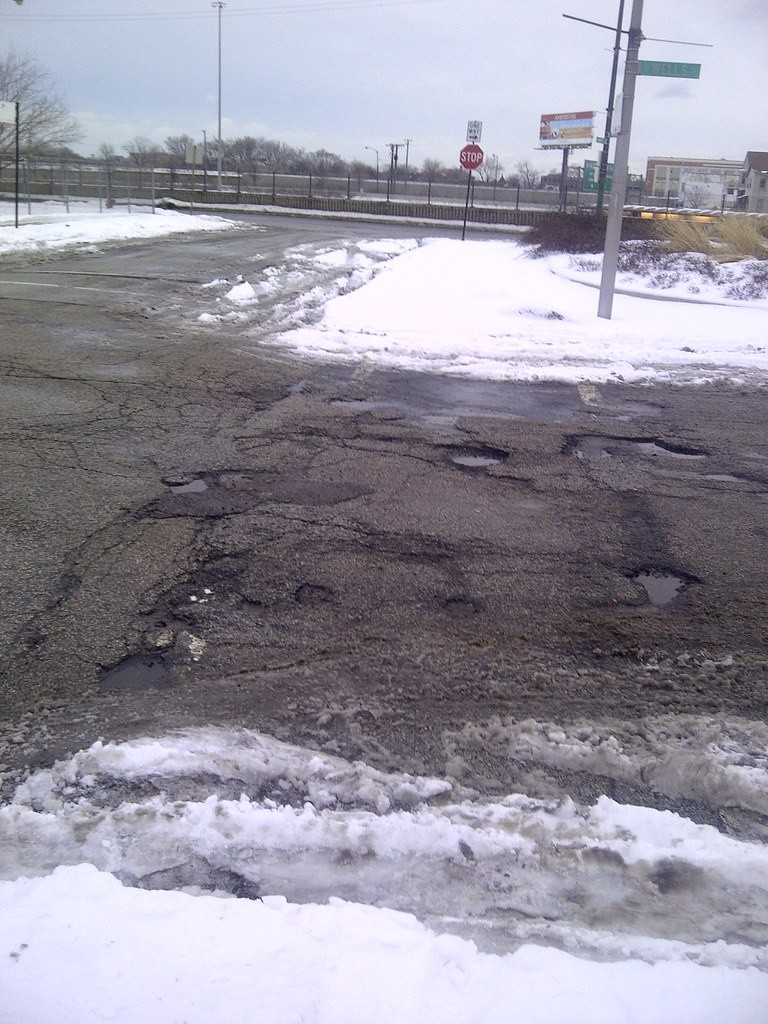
201,129,207,191
366,147,379,193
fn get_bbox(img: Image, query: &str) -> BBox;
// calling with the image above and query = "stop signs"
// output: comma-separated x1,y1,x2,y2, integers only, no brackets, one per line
460,144,484,170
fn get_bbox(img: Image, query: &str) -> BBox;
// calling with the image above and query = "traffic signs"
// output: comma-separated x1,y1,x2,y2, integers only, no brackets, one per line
582,158,630,196
